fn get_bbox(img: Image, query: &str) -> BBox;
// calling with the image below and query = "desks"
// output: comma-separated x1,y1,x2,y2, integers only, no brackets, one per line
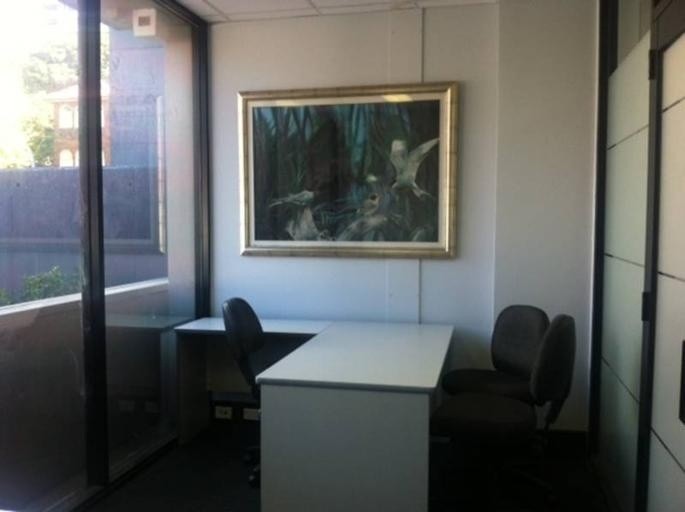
105,313,199,395
173,315,456,511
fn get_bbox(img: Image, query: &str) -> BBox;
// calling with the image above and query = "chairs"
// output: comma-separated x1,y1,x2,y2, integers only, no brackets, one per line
442,304,550,472
221,297,279,486
430,314,576,511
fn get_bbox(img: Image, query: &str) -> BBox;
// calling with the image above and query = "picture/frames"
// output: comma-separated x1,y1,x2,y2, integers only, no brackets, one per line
236,79,460,260
